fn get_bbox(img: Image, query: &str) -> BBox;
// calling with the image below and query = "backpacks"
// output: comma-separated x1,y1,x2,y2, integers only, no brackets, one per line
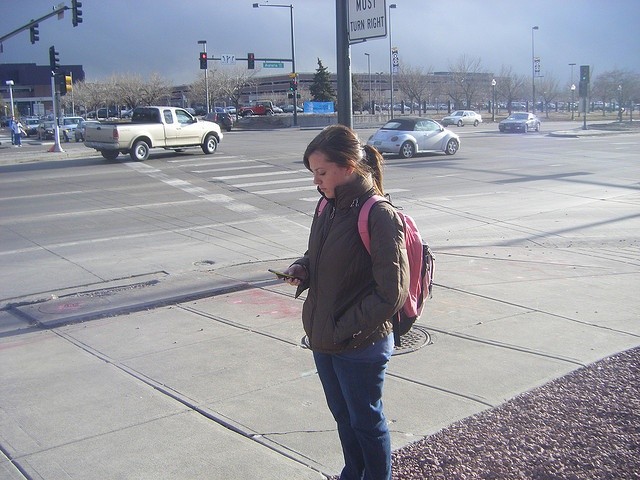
316,194,436,337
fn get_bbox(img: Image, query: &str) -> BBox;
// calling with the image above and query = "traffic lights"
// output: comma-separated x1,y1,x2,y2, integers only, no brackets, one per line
71,1,82,28
29,19,39,45
199,52,207,69
248,53,254,69
580,80,588,98
290,81,297,90
49,46,59,77
59,75,71,97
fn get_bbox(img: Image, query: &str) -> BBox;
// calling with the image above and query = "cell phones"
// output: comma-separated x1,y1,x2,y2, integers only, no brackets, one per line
268,269,302,280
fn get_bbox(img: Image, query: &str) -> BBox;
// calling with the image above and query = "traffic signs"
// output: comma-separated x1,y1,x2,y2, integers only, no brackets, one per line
348,0,387,44
221,54,236,64
263,62,285,68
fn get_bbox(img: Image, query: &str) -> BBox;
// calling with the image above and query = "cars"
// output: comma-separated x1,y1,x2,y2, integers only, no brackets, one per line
436,104,449,110
273,106,284,113
366,117,460,158
213,107,223,112
0,116,12,127
499,112,540,133
76,120,101,142
374,103,391,111
426,104,435,110
185,108,197,116
393,104,410,111
284,105,303,113
441,110,482,127
226,106,236,114
37,121,55,140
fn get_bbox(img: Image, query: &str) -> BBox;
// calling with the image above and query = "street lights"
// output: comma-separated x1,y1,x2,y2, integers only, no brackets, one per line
532,26,538,113
389,4,396,120
198,41,210,114
365,53,371,113
571,84,576,119
253,4,297,126
491,79,496,122
6,80,14,115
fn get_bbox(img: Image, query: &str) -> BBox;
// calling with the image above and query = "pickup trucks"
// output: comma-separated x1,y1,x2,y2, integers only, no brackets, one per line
84,105,223,161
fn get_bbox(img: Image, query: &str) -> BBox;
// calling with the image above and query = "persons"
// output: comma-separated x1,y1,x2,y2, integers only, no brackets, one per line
9,116,16,145
12,118,24,148
278,124,411,480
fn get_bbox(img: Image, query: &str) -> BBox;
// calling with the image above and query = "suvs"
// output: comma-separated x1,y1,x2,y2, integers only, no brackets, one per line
507,102,526,111
23,119,40,138
239,100,273,116
202,112,233,131
58,117,85,142
